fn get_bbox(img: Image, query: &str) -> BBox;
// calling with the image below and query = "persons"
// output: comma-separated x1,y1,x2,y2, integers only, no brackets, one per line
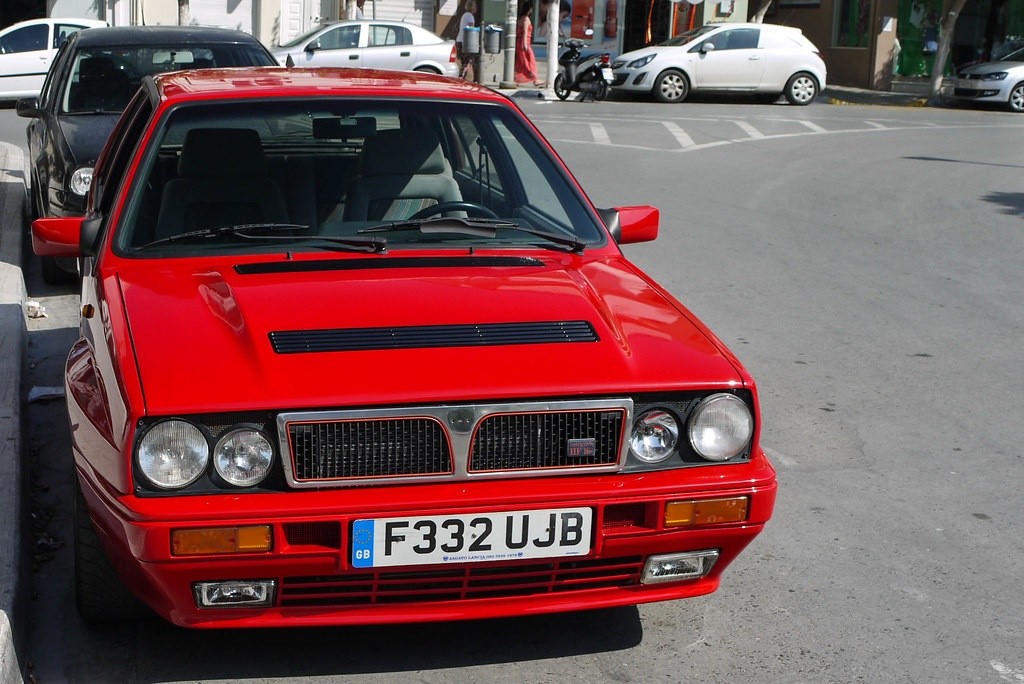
515,2,546,86
455,0,478,81
356,0,366,20
538,0,571,39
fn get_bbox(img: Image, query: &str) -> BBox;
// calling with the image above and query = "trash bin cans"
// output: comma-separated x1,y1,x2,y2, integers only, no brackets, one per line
462,27,480,54
485,24,502,54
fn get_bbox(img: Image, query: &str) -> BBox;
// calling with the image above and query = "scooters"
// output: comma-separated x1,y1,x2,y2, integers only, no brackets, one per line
552,28,615,101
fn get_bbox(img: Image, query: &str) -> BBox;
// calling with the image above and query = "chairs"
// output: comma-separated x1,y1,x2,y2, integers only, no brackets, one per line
180,58,215,71
152,127,296,243
71,57,138,110
341,127,469,223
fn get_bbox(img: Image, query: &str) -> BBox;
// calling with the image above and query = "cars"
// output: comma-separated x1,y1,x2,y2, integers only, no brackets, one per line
29,65,779,632
269,18,462,83
16,23,310,283
0,16,194,106
609,20,828,104
953,46,1024,112
